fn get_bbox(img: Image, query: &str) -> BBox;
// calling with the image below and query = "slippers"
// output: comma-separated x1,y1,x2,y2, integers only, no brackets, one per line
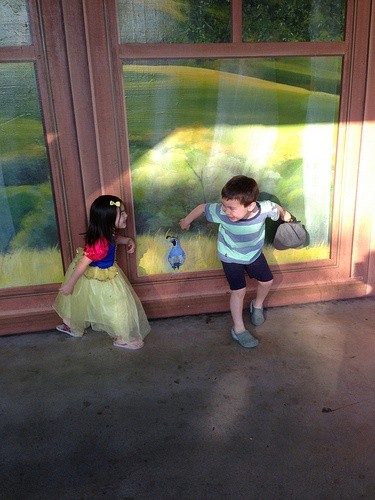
56,324,84,338
231,326,258,348
112,338,145,350
250,299,266,327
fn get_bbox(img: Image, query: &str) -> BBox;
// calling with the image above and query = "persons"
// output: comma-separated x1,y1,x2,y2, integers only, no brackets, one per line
178,175,293,348
53,194,151,350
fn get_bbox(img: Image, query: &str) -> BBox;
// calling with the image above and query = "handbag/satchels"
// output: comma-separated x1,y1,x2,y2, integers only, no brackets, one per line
274,220,307,251
